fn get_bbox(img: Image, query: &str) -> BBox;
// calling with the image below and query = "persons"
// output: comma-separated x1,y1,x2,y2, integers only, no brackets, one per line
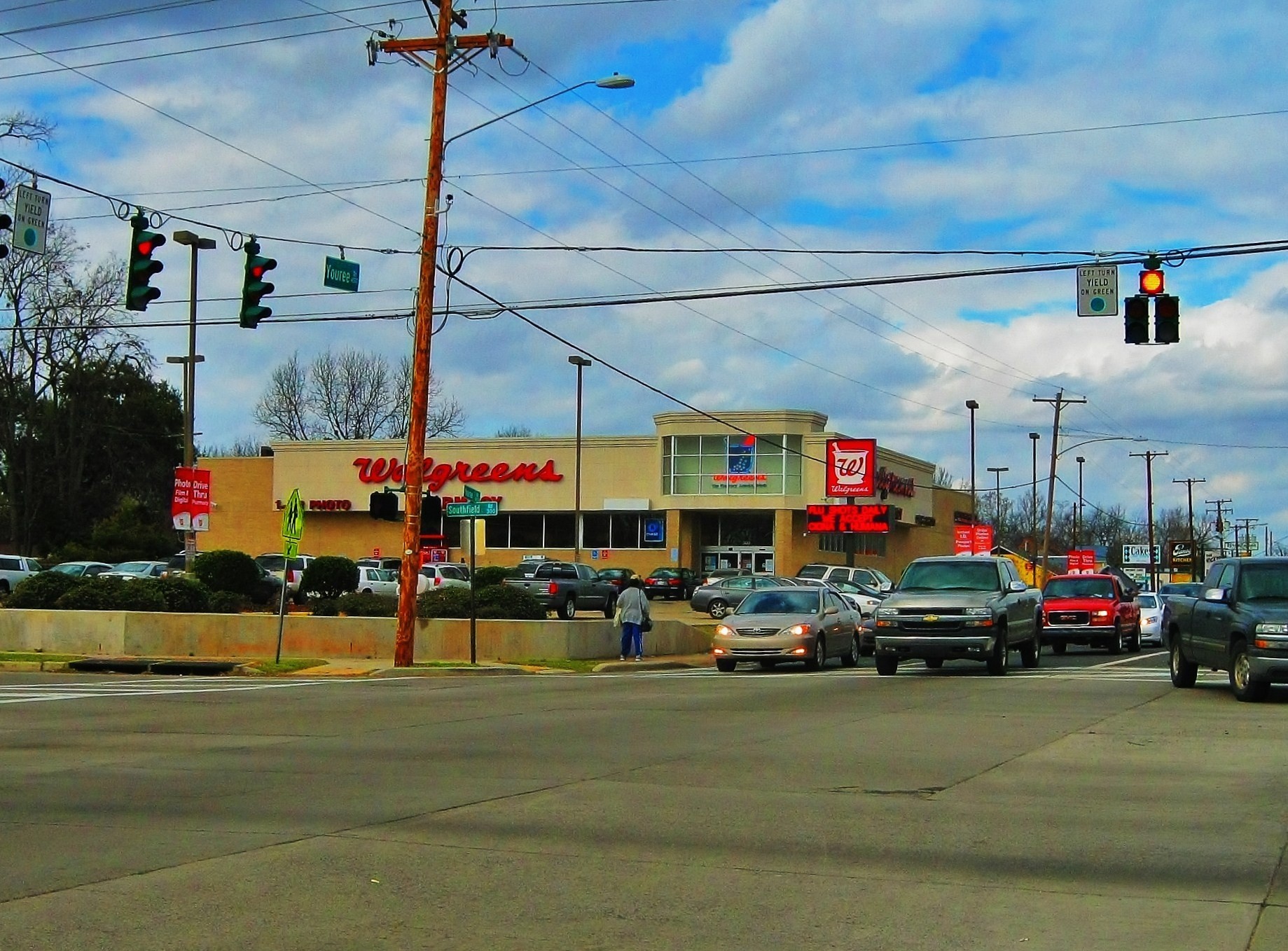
616,574,650,662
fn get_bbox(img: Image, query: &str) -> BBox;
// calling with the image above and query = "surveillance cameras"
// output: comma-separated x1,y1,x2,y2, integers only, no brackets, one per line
445,193,454,204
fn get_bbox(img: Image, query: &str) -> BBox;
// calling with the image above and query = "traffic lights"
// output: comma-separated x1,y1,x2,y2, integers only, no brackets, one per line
1124,297,1150,344
1139,270,1164,295
239,256,278,329
1154,296,1180,342
124,228,167,312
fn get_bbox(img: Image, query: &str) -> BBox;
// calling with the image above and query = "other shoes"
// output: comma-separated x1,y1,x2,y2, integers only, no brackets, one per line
635,655,642,661
619,654,627,661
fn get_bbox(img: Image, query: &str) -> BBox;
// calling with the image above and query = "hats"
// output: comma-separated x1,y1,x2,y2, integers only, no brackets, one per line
630,575,640,581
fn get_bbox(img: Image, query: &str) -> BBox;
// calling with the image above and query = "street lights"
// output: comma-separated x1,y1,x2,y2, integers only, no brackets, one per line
567,354,593,562
166,354,205,467
1028,432,1040,564
171,229,217,468
1076,456,1086,551
965,398,979,557
986,467,1010,557
391,74,637,665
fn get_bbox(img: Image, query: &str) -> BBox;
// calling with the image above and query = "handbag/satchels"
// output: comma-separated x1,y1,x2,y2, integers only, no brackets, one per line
641,617,653,632
613,607,622,628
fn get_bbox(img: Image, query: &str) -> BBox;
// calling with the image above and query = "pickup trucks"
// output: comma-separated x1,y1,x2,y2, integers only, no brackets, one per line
870,554,1045,677
1160,555,1288,704
1039,573,1143,657
499,560,619,620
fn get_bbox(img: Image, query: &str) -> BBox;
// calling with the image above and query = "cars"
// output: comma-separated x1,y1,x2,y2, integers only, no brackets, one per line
643,566,699,602
710,586,866,674
0,543,1216,658
